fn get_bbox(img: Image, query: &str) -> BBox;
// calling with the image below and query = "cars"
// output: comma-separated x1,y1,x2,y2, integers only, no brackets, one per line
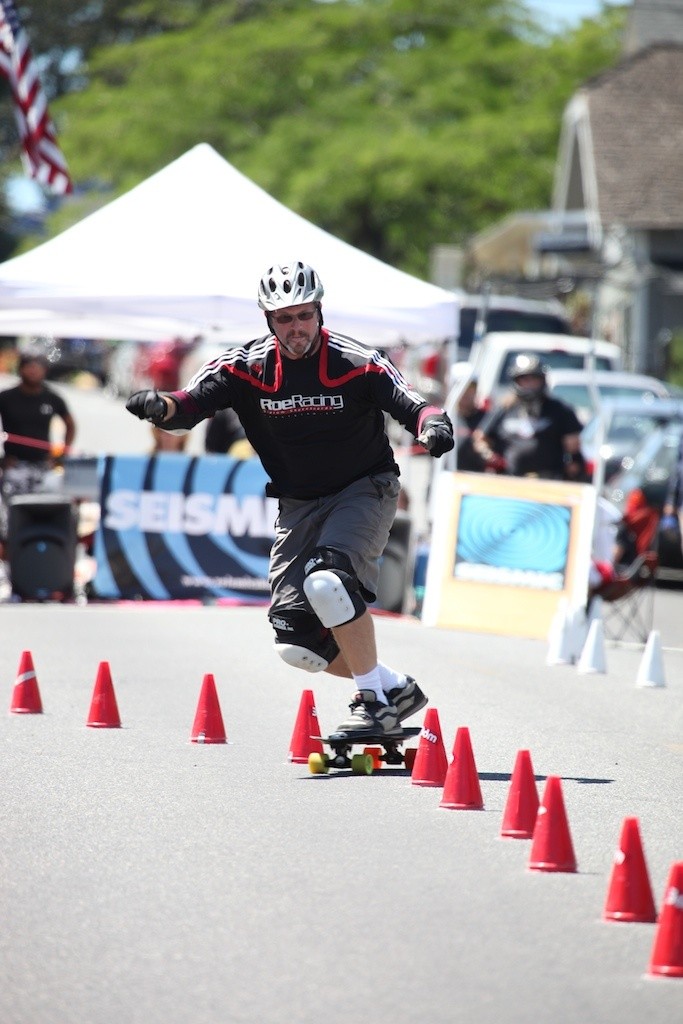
404,293,683,576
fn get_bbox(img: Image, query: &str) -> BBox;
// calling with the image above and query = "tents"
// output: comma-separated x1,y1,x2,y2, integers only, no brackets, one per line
0,140,460,615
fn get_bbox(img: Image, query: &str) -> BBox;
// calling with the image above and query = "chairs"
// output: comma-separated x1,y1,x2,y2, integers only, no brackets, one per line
589,473,672,642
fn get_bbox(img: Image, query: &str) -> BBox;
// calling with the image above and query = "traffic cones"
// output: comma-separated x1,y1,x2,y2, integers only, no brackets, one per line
187,673,231,745
604,816,659,923
435,724,489,811
408,707,452,789
530,776,582,874
283,689,331,764
498,748,544,840
647,862,683,980
12,650,45,716
84,658,124,729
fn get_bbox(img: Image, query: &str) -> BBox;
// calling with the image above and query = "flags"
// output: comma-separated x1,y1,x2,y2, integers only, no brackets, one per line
0,0,72,195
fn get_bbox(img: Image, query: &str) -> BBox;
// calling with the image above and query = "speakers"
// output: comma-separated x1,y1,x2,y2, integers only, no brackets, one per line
7,492,78,601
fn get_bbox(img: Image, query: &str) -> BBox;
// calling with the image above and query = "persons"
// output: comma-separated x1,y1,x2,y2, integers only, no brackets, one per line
125,259,455,739
0,349,75,468
458,352,588,481
137,337,244,453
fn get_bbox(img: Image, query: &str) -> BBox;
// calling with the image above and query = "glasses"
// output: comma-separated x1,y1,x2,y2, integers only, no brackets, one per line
266,306,318,325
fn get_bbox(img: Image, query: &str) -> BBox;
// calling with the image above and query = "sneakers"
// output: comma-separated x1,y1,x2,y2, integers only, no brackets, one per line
335,687,405,738
382,674,429,722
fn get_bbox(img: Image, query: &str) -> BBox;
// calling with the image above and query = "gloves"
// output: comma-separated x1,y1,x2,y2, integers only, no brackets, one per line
125,388,169,424
416,414,455,459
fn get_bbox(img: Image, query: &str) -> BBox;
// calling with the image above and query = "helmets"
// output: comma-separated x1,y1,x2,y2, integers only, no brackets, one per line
257,260,323,313
508,352,548,404
14,347,54,375
